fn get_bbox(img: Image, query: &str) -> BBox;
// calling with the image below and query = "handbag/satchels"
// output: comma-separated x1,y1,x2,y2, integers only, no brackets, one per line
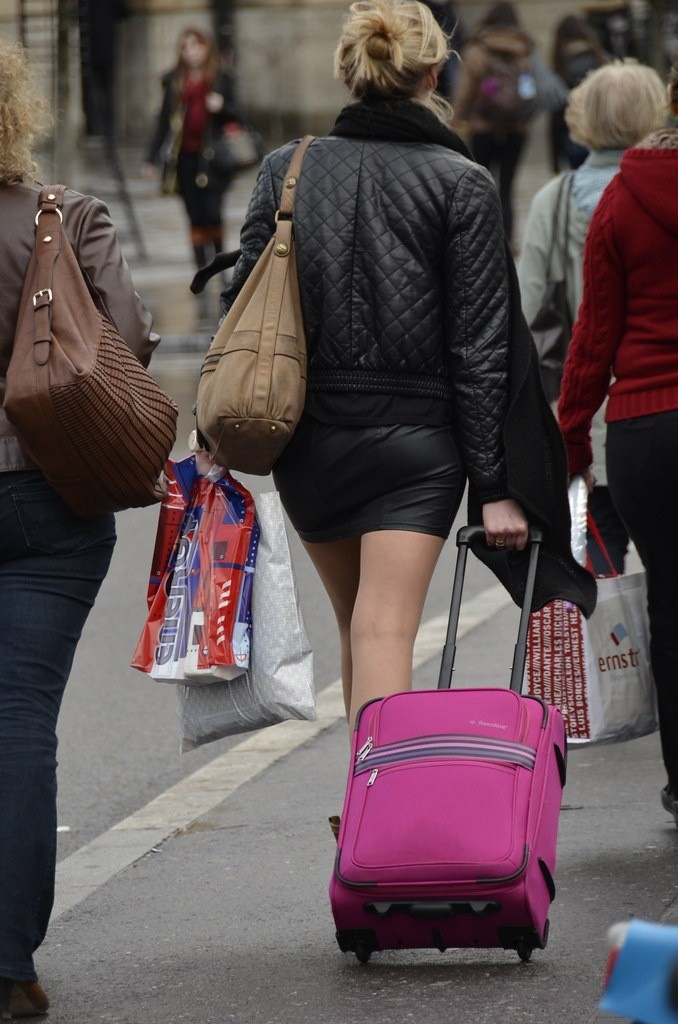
526,486,660,751
197,135,317,477
3,183,179,515
524,280,573,404
175,492,317,756
129,454,251,686
217,115,263,170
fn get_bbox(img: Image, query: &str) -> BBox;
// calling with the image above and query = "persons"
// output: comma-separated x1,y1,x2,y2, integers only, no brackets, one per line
0,48,160,1019
188,0,527,842
139,25,240,296
519,62,678,816
420,0,678,256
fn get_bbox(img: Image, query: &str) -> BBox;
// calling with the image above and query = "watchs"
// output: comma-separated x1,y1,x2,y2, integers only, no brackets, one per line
188,430,208,452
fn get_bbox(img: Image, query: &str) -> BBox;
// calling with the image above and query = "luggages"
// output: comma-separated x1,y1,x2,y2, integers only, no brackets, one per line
327,523,567,964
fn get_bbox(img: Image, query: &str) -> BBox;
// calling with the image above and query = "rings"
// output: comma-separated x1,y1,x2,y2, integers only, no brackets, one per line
495,540,505,548
